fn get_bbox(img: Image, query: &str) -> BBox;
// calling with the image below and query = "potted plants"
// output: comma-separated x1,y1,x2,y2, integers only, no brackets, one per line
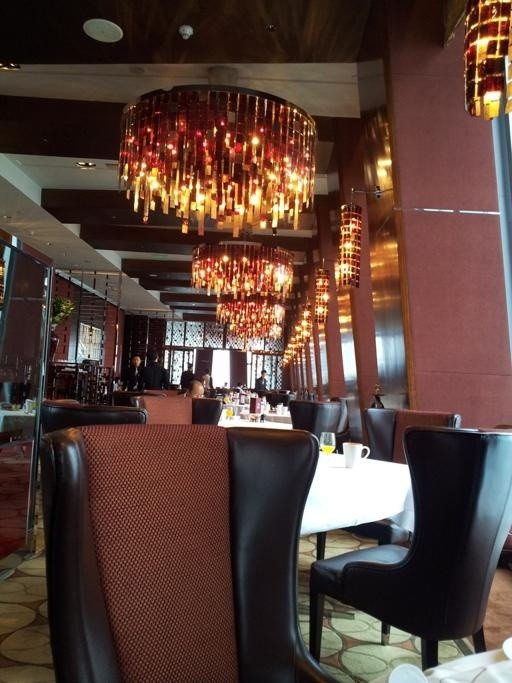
41,293,75,362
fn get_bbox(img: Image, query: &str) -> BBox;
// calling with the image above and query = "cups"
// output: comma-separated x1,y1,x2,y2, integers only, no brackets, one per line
22,399,35,413
343,441,371,469
223,384,289,424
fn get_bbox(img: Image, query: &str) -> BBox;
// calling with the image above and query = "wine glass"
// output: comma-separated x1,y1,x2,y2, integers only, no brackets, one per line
319,431,336,466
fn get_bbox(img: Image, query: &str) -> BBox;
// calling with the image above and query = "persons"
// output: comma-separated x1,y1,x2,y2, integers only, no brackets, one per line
181,378,205,399
181,362,196,389
254,369,268,391
202,368,212,389
235,382,247,394
224,381,229,388
137,350,171,389
127,354,147,391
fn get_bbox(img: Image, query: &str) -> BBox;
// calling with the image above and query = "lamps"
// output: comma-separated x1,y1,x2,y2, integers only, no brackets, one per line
336,183,382,290
279,254,334,369
116,69,317,238
464,0,512,121
190,239,295,342
